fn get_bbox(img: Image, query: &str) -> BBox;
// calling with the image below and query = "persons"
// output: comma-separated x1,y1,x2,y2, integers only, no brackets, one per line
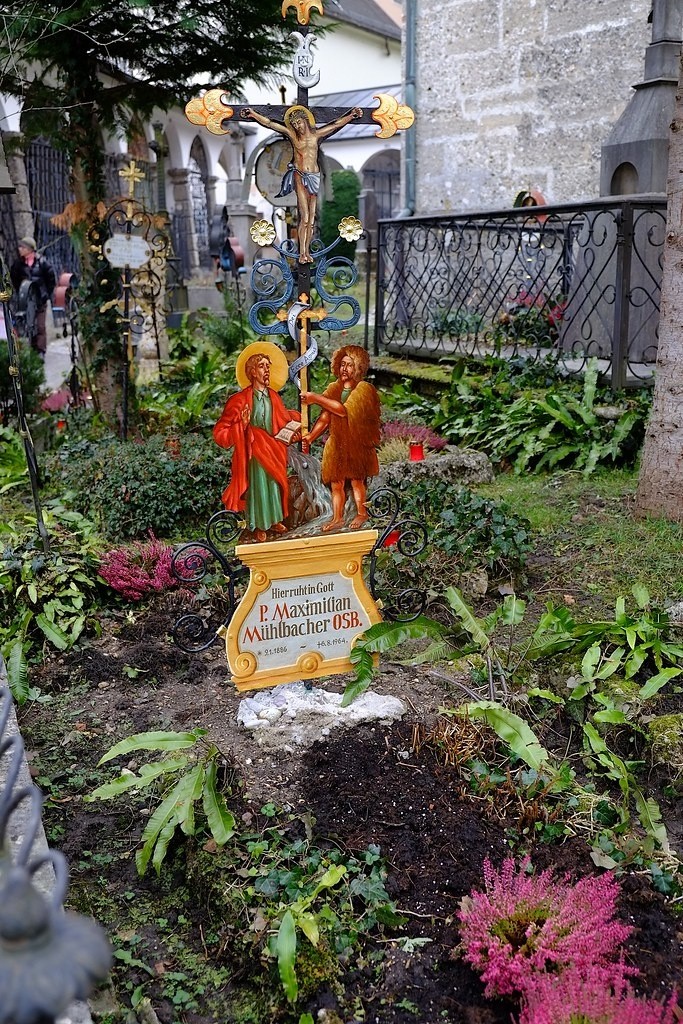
216,262,232,290
8,238,55,365
241,107,363,264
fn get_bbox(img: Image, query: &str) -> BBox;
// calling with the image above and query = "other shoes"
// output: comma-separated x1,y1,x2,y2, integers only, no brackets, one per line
37,351,44,359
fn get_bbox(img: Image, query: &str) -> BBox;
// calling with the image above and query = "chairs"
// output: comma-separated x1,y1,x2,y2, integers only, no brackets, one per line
52,272,76,314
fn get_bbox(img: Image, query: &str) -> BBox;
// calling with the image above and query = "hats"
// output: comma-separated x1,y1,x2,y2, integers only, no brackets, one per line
17,236,37,250
